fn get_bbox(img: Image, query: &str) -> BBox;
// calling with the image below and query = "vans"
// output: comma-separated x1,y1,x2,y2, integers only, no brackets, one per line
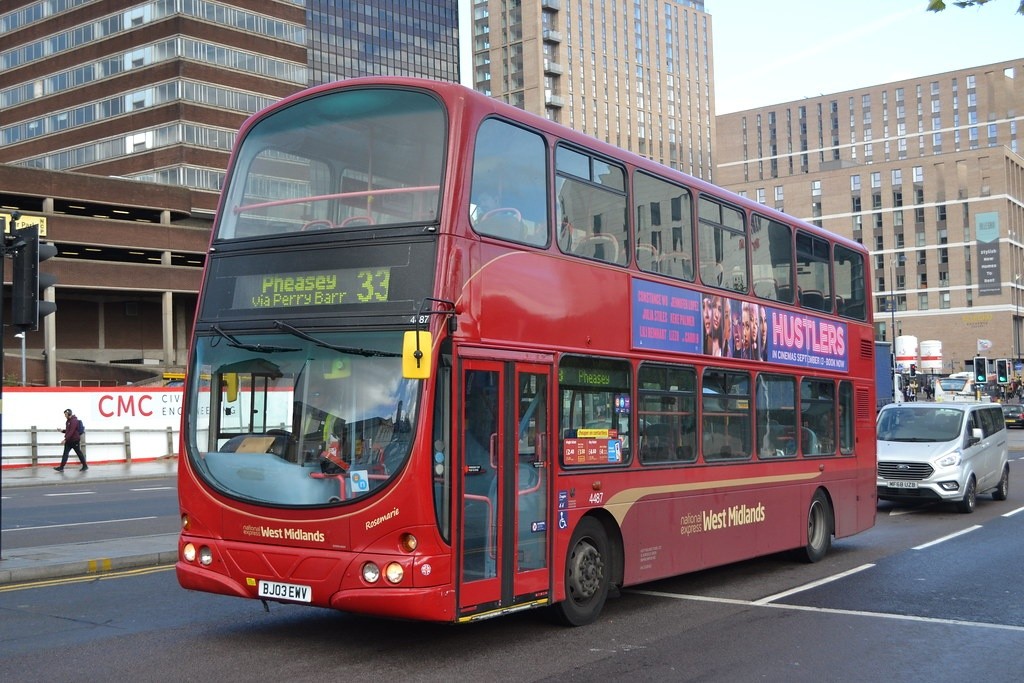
876,402,1009,514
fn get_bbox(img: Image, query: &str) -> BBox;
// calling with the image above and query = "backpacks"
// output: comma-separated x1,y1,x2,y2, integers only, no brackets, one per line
77,420,85,435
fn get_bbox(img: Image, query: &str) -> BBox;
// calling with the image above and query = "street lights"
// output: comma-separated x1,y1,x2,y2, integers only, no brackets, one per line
1015,272,1024,358
889,259,897,354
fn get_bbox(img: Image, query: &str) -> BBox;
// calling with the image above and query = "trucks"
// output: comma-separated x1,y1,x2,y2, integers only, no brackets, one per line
874,341,905,414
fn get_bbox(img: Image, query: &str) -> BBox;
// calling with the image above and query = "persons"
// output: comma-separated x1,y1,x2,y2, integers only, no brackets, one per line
702,293,769,361
292,381,346,459
53,409,88,472
470,191,499,227
819,403,846,447
923,382,932,400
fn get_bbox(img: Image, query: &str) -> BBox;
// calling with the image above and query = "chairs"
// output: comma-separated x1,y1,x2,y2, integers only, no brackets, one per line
475,207,862,319
641,424,674,450
785,428,817,454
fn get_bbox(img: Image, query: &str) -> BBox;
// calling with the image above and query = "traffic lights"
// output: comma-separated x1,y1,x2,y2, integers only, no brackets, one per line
910,364,916,376
11,223,57,332
996,359,1009,384
974,357,987,383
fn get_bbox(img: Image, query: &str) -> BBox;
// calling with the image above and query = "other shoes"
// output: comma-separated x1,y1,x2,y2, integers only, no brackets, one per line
79,466,88,471
52,466,64,472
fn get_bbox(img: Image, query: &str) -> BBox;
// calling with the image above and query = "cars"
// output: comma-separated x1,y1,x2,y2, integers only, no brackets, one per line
1002,404,1024,429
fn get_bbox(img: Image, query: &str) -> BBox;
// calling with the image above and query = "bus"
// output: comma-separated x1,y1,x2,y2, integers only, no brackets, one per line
935,372,1001,402
169,72,879,628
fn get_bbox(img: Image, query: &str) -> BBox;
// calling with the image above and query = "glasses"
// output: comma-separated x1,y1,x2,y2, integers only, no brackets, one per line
309,392,321,397
